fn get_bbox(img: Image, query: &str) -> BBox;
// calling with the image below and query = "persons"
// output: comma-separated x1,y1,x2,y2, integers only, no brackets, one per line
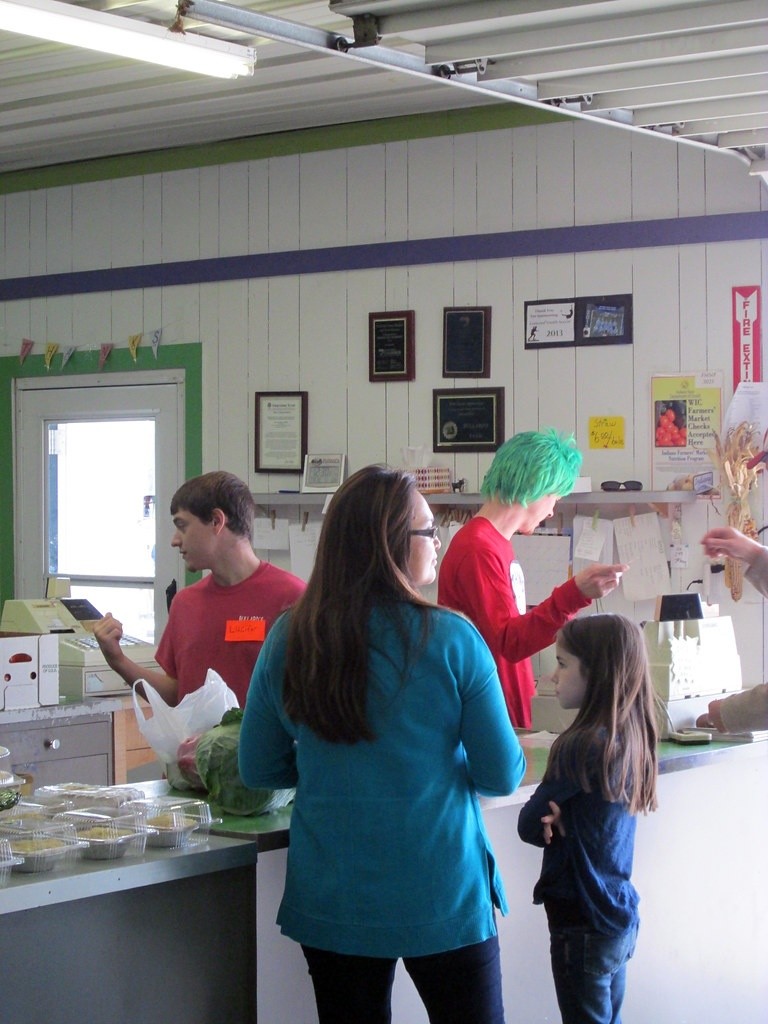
237,463,527,1023
518,614,659,1024
93,469,309,711
700,524,768,735
437,425,630,730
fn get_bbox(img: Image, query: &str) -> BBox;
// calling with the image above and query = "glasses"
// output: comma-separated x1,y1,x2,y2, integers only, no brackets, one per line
409,527,439,538
602,481,643,491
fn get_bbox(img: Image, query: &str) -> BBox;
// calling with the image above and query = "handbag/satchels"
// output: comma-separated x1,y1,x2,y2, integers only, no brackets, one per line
134,668,242,790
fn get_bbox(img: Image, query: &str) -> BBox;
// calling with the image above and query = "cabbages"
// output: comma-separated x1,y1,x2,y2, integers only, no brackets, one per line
195,706,297,817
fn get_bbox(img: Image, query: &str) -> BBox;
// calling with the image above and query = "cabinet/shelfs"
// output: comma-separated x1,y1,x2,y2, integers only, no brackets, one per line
114,708,159,785
249,488,699,505
1,796,262,1024
0,703,114,796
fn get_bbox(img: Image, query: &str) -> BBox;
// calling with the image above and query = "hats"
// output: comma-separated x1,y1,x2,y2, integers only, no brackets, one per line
747,428,768,470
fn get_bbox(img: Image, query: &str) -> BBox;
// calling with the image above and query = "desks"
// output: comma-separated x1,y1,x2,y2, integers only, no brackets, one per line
110,728,767,851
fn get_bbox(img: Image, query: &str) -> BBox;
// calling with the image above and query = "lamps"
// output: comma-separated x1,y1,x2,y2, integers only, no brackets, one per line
2,0,258,80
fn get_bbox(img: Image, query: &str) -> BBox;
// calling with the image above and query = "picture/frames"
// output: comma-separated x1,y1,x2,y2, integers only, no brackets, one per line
254,391,308,473
432,387,505,453
367,309,417,383
441,305,493,378
523,293,634,350
301,453,345,493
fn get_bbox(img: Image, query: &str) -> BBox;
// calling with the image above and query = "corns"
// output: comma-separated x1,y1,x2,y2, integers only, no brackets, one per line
724,498,759,601
